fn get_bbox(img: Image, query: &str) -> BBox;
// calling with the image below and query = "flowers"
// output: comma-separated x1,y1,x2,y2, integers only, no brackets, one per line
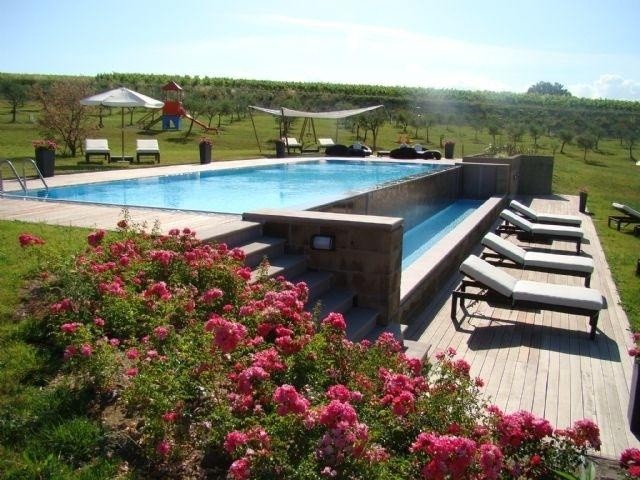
200,135,216,149
627,325,640,363
578,185,588,193
32,138,63,153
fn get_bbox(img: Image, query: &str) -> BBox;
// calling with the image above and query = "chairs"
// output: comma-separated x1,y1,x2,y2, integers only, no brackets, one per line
85,138,111,164
281,138,441,159
495,209,584,254
509,199,582,227
451,255,603,341
608,202,640,231
136,139,161,164
480,232,594,288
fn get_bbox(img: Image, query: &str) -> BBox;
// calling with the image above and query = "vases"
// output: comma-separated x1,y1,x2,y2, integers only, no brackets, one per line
445,144,455,159
579,192,587,212
35,148,55,178
627,363,640,432
199,144,211,163
276,142,285,158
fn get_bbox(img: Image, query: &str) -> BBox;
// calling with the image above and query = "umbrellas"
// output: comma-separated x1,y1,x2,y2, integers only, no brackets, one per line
78,86,165,161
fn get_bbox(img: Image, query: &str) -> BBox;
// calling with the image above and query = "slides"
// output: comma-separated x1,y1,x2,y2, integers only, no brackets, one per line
179,107,218,130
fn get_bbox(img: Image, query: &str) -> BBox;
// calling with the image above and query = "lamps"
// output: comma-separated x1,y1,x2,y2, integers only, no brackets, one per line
310,234,336,251
